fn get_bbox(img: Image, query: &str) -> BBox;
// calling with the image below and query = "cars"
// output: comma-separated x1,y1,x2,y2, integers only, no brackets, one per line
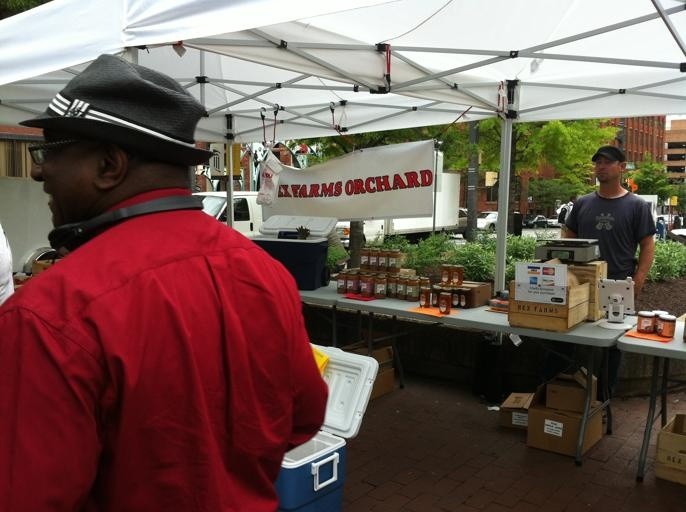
546,215,565,228
655,213,681,226
524,214,548,230
446,207,468,240
477,210,498,233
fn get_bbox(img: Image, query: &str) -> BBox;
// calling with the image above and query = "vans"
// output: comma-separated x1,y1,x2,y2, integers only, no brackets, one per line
192,190,264,238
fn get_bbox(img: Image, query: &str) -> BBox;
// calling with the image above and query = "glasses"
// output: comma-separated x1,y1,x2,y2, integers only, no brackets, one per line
29,139,78,165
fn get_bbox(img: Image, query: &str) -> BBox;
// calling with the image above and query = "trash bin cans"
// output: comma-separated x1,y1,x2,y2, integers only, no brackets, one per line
508,213,523,236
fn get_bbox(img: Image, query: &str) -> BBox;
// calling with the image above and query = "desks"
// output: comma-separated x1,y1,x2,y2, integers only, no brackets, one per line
299,281,364,364
336,298,640,465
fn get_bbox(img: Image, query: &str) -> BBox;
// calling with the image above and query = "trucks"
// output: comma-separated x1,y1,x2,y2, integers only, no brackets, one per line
334,172,461,247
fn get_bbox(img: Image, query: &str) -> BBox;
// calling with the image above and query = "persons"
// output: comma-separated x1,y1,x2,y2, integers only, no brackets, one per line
1,52,330,512
558,194,576,237
559,143,656,396
673,211,682,242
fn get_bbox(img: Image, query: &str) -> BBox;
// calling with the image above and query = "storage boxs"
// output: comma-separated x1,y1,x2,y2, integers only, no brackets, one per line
339,340,395,400
653,413,686,485
617,320,686,483
526,395,604,456
508,280,590,331
501,392,538,427
547,369,599,411
533,259,607,320
242,217,337,289
434,280,490,307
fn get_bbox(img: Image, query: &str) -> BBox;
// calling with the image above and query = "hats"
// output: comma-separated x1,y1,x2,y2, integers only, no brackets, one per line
18,54,214,166
592,147,625,162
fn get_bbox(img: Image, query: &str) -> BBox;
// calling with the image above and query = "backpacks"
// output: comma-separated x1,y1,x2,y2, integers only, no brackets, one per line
558,202,573,223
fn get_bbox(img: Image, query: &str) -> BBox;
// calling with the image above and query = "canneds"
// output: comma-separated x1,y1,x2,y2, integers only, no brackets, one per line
657,313,677,338
636,309,655,334
653,310,668,333
337,247,471,315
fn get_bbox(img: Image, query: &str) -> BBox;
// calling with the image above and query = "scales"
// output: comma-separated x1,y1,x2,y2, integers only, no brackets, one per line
535,239,601,265
